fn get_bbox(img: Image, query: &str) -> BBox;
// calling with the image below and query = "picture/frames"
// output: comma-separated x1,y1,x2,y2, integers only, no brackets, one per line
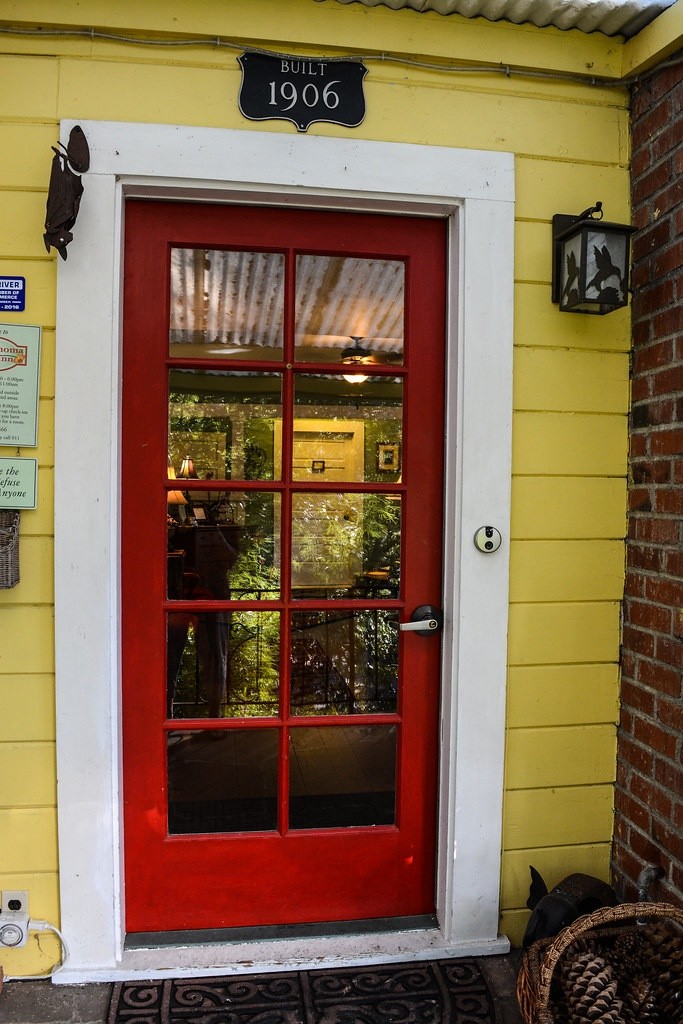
376,441,402,472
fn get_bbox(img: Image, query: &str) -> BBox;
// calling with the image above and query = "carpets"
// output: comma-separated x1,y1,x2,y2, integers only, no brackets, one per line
105,956,501,1024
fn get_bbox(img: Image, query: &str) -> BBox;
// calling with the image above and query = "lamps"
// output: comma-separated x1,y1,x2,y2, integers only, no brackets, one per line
176,453,199,525
341,336,372,387
552,202,639,318
167,464,189,506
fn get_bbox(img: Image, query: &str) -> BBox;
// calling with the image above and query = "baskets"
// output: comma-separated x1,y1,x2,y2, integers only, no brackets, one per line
0,510,20,589
516,903,683,1024
